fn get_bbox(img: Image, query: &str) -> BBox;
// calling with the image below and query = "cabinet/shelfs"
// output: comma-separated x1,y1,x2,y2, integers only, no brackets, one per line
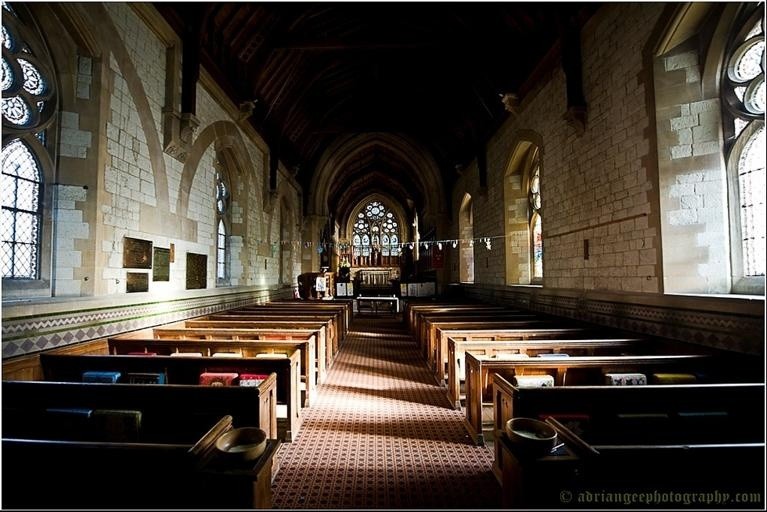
387,279,438,300
356,297,399,314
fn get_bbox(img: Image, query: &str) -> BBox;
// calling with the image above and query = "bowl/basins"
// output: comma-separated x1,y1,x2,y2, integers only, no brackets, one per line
215,426,267,462
505,417,556,452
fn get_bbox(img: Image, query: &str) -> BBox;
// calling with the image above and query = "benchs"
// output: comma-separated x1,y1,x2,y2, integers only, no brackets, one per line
245,299,354,341
434,328,587,387
446,335,643,409
492,364,765,491
40,353,304,444
409,306,540,357
2,441,277,512
153,321,329,383
210,311,339,362
425,320,583,377
108,336,317,442
464,350,712,446
4,378,279,497
220,310,344,359
